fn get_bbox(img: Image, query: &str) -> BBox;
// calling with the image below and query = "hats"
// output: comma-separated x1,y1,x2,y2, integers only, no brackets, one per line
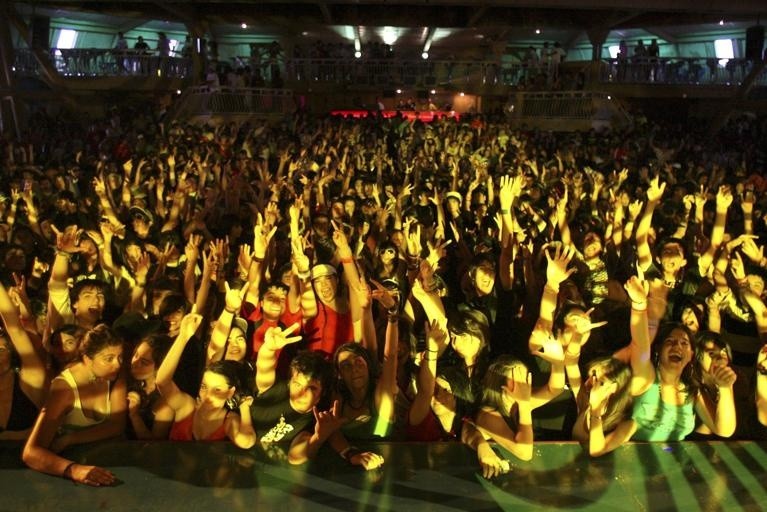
446,191,463,203
129,206,154,222
309,264,336,280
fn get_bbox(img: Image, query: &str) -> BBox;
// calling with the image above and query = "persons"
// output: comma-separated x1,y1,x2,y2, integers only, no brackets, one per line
1,30,766,486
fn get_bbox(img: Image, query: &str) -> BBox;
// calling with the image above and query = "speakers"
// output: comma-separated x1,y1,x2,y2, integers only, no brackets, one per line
32,15,50,49
745,25,765,62
